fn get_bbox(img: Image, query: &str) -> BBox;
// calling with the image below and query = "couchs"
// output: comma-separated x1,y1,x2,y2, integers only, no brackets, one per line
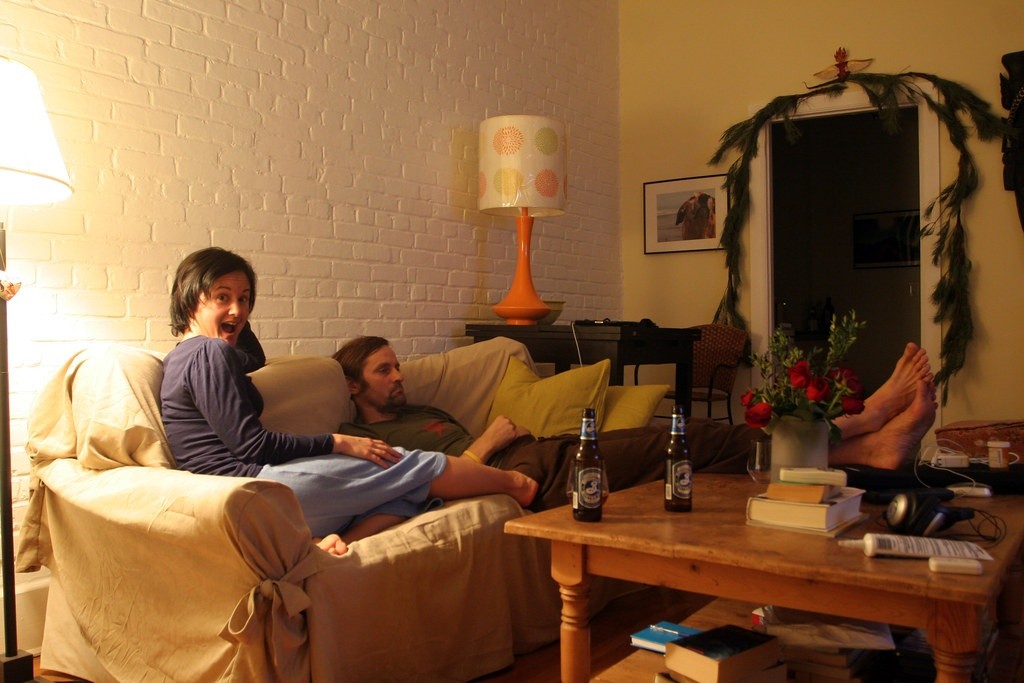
28,332,672,683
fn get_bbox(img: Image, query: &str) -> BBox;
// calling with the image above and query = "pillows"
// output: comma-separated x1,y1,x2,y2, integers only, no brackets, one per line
596,384,671,435
485,355,610,445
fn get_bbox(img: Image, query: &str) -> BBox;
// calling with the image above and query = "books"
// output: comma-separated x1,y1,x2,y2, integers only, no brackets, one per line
628,604,899,683
744,477,866,539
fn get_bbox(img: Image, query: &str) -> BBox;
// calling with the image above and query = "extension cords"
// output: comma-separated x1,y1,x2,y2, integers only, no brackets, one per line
935,456,971,468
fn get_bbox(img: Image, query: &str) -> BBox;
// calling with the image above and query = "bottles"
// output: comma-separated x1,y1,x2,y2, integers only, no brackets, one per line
665,406,693,512
572,409,604,522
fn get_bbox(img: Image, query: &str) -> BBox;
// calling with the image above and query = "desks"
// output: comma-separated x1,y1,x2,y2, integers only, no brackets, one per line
464,319,701,424
506,468,1024,683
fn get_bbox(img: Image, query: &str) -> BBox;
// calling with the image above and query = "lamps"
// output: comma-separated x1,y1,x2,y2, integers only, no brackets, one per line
476,113,567,328
0,55,77,683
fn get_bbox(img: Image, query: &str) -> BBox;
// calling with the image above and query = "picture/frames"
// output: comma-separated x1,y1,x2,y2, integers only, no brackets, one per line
643,173,732,254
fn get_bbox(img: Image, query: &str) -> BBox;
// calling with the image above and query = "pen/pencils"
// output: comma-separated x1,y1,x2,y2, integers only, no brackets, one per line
650,625,690,637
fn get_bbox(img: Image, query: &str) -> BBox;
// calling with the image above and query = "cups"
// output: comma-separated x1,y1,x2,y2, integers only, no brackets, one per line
987,441,1020,468
747,439,772,486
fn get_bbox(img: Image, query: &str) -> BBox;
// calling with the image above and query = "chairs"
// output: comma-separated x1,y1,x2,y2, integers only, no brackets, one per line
634,323,749,429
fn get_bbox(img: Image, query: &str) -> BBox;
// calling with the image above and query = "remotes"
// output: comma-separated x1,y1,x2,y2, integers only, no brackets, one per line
779,467,847,487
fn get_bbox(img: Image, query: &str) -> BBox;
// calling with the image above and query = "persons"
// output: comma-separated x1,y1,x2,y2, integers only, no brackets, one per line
331,335,939,513
158,247,540,558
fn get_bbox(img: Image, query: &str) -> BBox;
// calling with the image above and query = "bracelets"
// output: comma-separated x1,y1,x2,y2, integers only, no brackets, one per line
462,449,486,466
